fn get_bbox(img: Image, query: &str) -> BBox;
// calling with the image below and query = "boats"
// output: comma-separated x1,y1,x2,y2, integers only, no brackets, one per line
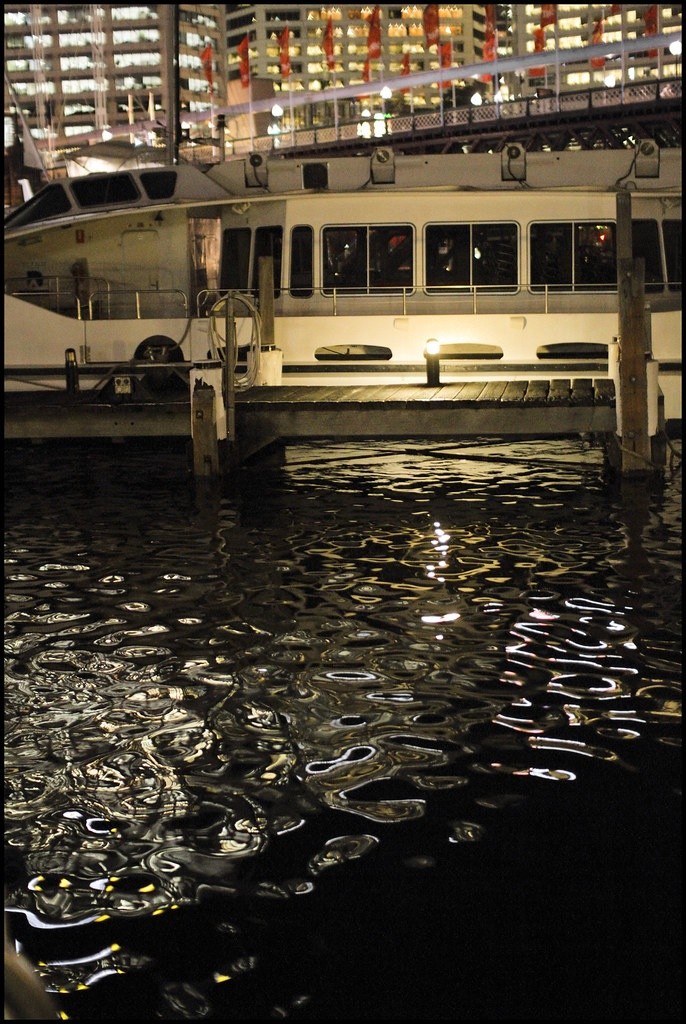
3,185,686,423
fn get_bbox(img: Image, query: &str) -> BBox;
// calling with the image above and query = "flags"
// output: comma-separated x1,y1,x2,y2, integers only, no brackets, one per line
277,23,290,78
612,4,621,15
319,15,335,72
356,5,381,100
235,36,249,88
480,5,496,83
399,51,410,94
642,4,659,58
436,40,452,89
527,28,545,77
199,44,213,97
537,4,556,31
423,5,440,48
590,19,605,68
